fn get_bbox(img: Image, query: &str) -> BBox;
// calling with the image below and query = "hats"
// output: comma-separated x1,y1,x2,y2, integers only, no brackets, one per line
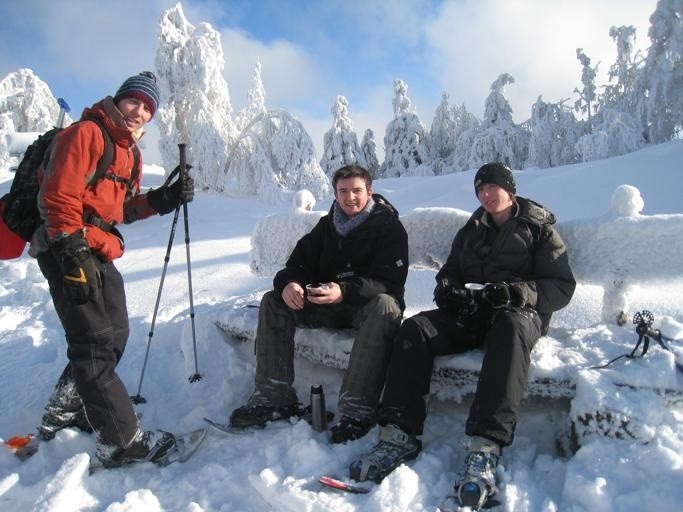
113,71,161,123
474,163,516,197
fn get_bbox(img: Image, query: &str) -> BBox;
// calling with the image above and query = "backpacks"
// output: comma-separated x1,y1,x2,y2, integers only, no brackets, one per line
2,117,141,243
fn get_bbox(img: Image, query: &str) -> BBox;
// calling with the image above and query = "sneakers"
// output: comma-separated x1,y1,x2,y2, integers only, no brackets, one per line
96,430,176,469
38,407,94,441
230,404,299,426
350,423,422,484
328,415,376,443
454,437,503,512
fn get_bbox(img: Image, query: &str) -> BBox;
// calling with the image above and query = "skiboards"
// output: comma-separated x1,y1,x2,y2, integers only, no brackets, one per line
320,443,505,512
202,404,323,438
9,416,206,475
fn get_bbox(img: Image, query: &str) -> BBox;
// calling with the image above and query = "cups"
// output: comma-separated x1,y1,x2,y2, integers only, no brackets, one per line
304,280,330,298
465,280,486,305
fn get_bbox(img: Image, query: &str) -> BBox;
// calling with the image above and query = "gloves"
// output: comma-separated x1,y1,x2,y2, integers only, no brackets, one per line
147,173,195,216
437,285,478,319
50,227,103,305
484,281,518,309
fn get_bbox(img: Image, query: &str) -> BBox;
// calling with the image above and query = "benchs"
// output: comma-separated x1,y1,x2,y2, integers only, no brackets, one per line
214,185,683,456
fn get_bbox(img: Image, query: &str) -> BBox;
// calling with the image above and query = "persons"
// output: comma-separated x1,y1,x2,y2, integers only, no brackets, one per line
349,163,575,511
228,164,408,444
27,71,195,468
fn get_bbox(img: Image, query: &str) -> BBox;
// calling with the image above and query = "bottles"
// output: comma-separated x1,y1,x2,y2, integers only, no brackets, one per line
310,383,326,431
